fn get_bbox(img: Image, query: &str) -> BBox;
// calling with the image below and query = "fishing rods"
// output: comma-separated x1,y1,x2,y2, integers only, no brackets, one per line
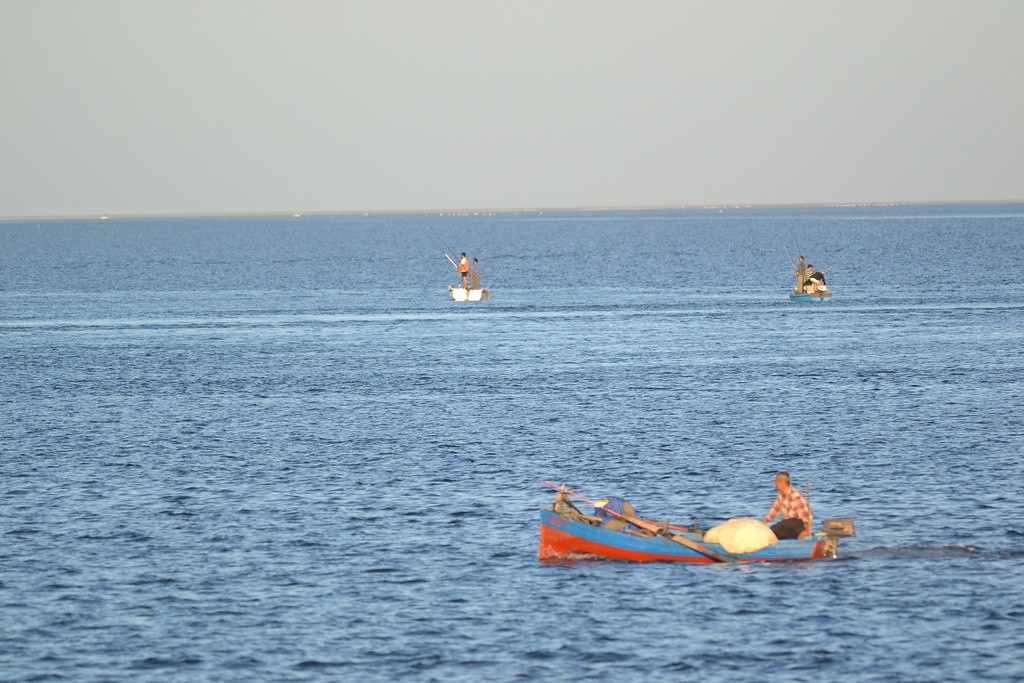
821,265,832,275
422,227,472,277
782,233,801,277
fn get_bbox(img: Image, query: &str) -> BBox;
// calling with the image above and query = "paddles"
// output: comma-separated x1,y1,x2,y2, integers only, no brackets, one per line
543,479,741,563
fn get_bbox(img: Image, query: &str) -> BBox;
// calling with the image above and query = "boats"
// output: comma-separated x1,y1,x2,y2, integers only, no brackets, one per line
538,475,856,564
445,283,489,301
784,287,832,303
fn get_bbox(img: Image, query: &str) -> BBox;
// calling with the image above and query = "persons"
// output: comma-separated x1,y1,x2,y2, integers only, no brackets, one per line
804,264,825,285
793,256,806,294
760,471,814,540
468,258,481,290
456,252,469,290
